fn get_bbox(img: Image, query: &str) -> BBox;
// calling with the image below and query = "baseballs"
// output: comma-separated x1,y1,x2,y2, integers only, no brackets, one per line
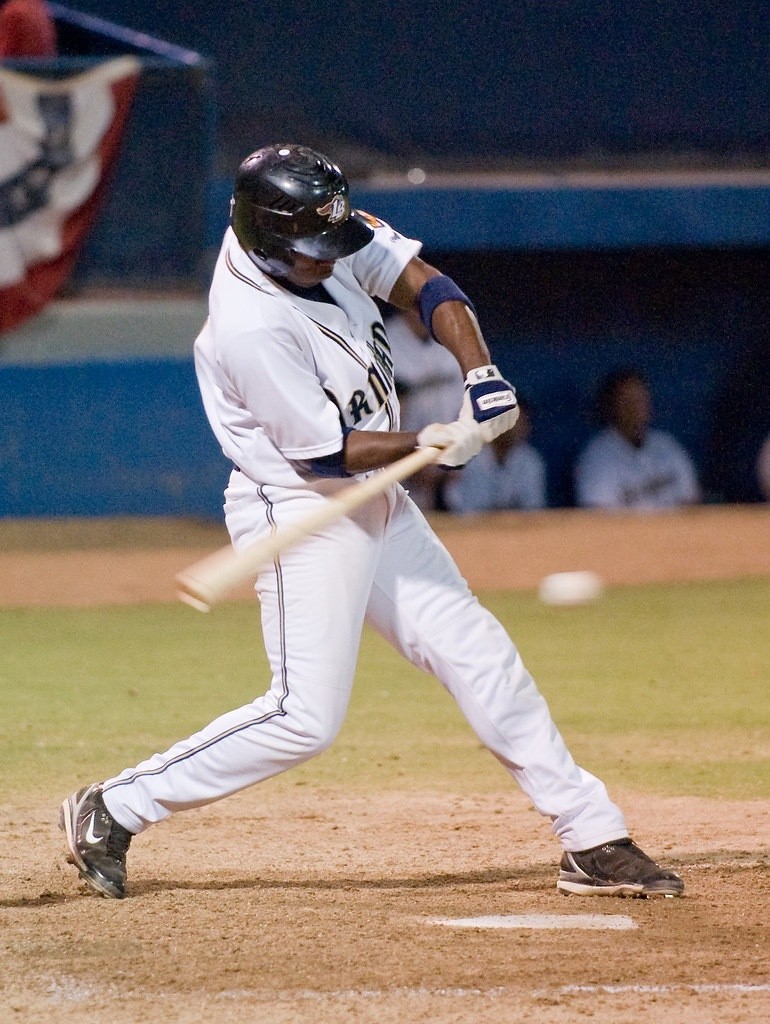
538,569,596,606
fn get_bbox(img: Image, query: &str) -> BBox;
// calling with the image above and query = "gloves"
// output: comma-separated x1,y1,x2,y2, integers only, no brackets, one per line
459,364,522,443
417,420,483,471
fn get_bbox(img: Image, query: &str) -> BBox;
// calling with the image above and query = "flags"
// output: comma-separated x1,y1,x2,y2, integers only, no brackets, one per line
0,54,141,340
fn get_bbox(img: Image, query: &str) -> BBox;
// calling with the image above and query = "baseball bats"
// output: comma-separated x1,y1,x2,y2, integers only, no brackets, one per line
174,441,443,616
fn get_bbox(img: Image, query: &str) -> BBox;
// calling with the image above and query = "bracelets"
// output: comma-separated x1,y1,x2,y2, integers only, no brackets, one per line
413,276,476,343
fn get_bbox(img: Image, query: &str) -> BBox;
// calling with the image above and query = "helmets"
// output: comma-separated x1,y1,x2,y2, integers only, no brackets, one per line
231,144,375,277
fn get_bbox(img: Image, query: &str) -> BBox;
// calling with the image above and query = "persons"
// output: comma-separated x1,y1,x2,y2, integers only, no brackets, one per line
384,311,545,512
576,372,703,507
58,144,685,898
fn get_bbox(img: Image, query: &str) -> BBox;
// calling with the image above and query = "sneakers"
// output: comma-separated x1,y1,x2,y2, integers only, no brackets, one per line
61,782,135,898
557,838,684,898
